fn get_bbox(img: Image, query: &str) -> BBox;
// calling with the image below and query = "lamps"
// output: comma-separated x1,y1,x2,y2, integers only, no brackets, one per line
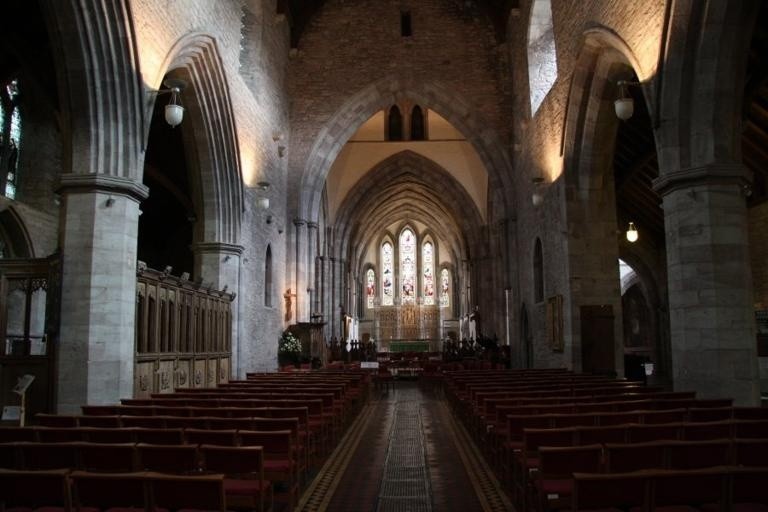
247,180,271,212
272,133,289,158
624,220,640,244
611,79,643,122
148,86,185,128
529,182,554,208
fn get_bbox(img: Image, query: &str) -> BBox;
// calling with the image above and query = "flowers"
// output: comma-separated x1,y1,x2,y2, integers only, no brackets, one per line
279,331,302,352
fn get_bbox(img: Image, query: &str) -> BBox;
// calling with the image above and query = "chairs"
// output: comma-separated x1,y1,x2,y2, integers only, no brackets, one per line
366,350,453,393
